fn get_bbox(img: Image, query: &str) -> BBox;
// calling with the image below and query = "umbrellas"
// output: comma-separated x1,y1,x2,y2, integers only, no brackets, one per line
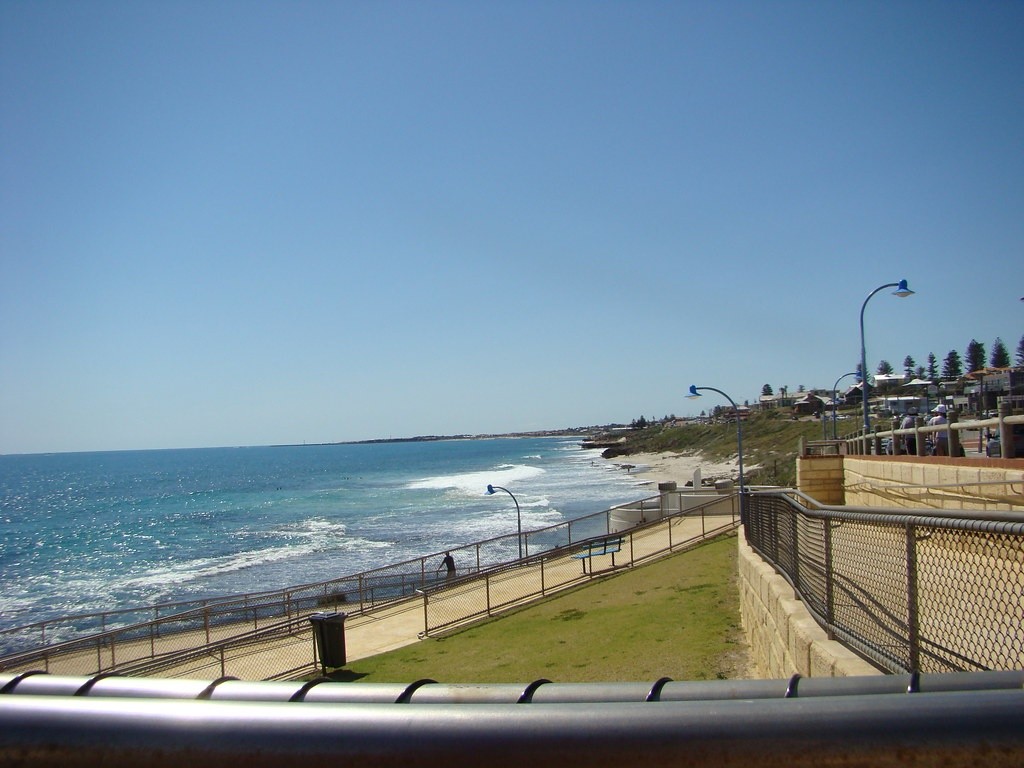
902,379,933,387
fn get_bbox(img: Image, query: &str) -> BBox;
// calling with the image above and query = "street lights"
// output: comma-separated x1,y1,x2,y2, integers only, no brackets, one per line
484,485,523,569
683,385,745,528
824,399,843,441
858,279,916,456
831,365,862,439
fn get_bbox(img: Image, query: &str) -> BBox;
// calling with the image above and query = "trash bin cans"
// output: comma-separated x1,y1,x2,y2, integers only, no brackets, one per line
308,610,348,669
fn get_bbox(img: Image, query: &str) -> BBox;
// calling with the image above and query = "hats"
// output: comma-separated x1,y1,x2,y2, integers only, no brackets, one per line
936,405,946,413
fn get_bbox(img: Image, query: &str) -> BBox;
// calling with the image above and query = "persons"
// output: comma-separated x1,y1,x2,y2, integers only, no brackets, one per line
899,404,947,456
438,552,456,578
